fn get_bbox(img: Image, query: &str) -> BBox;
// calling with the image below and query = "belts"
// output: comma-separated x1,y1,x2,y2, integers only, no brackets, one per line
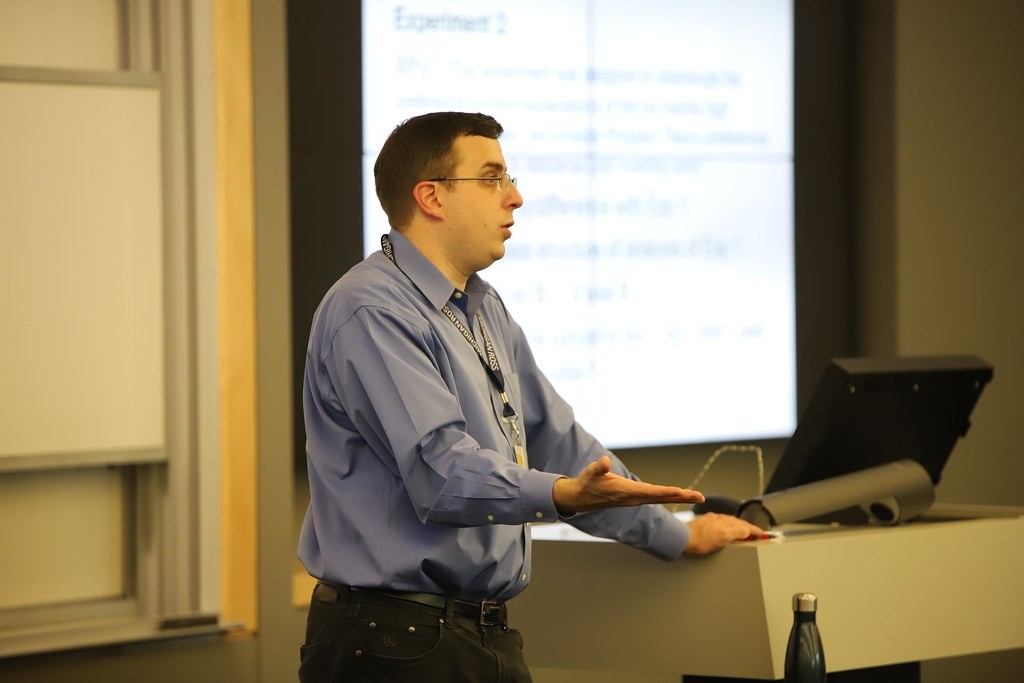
316,580,506,627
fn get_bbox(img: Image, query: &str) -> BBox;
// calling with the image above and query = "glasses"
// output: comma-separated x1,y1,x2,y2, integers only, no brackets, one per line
427,173,518,192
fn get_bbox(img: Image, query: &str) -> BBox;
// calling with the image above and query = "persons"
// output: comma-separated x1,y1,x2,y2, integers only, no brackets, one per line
298,111,763,681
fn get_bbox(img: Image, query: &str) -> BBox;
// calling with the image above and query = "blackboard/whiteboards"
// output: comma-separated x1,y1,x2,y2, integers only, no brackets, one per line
1,65,170,473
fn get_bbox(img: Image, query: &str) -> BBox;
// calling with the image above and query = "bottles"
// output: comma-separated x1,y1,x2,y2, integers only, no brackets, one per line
785,592,829,681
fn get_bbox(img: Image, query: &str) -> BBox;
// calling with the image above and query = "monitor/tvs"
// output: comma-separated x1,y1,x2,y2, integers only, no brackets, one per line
764,355,996,500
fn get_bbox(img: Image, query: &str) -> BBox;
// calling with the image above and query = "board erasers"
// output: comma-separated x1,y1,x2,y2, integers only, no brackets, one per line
159,616,219,630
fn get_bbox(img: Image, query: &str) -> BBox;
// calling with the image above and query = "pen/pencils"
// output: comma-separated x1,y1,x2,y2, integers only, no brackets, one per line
738,535,777,541
